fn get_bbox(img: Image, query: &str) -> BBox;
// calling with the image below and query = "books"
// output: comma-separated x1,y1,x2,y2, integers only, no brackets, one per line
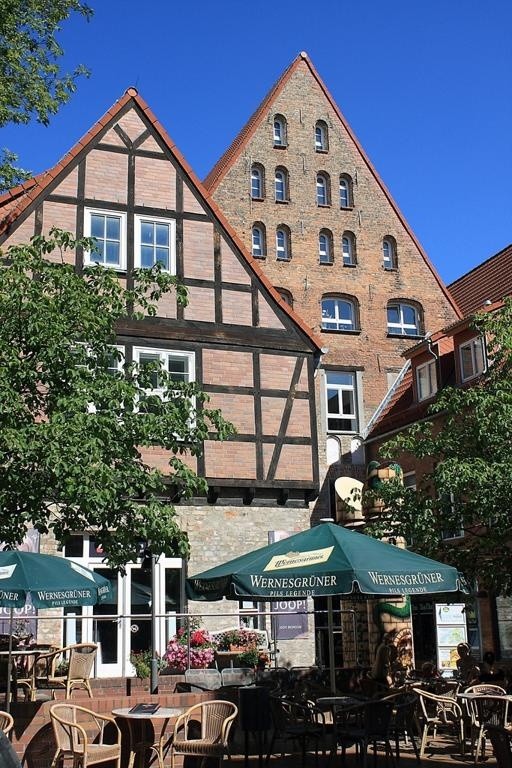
128,702,161,715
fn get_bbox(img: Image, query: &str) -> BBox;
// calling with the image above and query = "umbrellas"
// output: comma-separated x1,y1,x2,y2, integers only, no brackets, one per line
186,519,470,695
0,548,112,714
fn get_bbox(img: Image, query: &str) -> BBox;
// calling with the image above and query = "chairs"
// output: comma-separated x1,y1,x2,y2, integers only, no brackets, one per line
1,632,512,767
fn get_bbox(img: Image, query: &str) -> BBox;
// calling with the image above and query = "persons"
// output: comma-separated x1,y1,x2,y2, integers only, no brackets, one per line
417,641,512,722
373,638,390,690
